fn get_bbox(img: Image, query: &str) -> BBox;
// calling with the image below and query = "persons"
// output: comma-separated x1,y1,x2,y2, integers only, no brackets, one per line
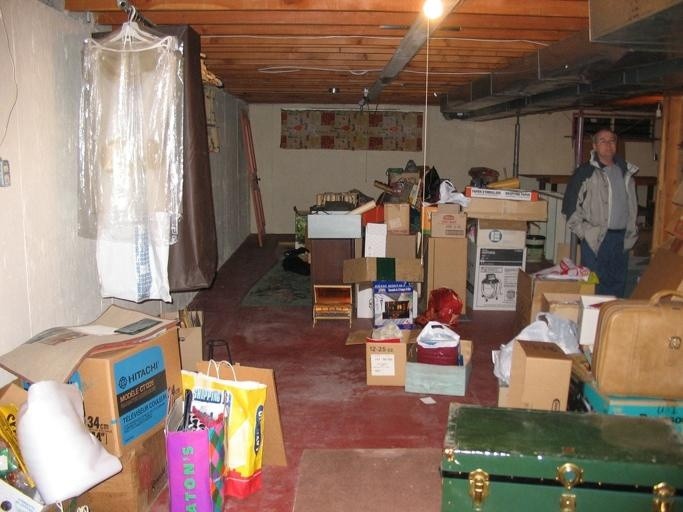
561,121,638,299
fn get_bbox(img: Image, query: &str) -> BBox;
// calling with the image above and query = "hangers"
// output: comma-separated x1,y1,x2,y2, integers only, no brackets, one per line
83,4,177,54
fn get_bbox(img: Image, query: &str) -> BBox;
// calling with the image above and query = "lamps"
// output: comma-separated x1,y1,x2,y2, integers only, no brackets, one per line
422,0,444,19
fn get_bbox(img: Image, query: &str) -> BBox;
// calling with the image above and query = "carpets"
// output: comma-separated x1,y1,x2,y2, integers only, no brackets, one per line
239,259,312,306
292,448,443,512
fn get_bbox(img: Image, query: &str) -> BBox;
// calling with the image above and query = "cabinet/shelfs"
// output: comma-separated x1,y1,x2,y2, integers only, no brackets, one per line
204,87,249,270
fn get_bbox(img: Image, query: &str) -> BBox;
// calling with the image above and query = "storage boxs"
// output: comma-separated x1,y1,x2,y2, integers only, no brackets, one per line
443,400,682,511
404,338,473,396
307,199,620,351
73,425,168,511
345,329,430,386
19,327,184,458
509,339,574,412
564,352,593,400
580,381,683,434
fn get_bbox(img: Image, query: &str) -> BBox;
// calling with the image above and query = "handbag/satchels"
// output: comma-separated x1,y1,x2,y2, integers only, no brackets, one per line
283,247,311,275
163,359,267,511
590,290,683,399
